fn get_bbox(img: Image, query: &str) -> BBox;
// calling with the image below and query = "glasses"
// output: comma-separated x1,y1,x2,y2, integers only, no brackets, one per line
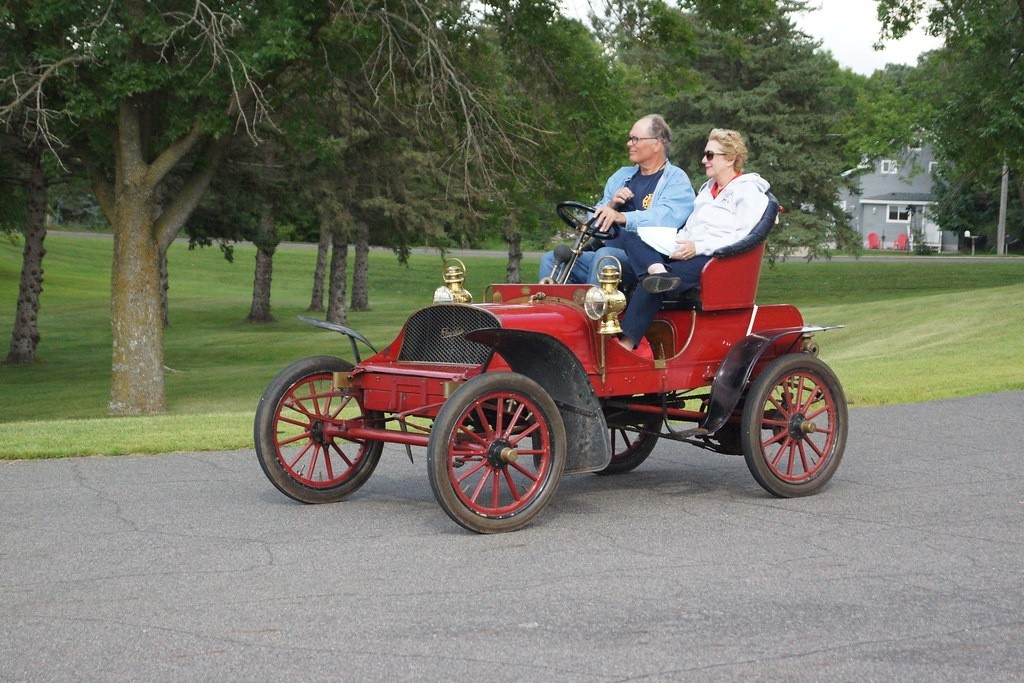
627,136,658,145
701,150,728,161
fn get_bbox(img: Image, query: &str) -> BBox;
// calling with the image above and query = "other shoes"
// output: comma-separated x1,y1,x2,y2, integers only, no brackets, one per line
641,272,682,293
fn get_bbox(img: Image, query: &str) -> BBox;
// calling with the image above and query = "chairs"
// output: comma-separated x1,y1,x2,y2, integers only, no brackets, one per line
868,233,880,250
894,234,907,251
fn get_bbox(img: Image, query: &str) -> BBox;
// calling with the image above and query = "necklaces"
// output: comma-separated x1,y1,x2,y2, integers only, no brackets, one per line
657,162,666,172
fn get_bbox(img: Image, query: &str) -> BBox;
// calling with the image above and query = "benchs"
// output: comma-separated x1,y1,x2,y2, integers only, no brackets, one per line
660,190,778,311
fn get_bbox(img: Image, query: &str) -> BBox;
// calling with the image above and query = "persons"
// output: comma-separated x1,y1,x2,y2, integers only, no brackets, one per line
617,128,771,351
538,114,696,286
